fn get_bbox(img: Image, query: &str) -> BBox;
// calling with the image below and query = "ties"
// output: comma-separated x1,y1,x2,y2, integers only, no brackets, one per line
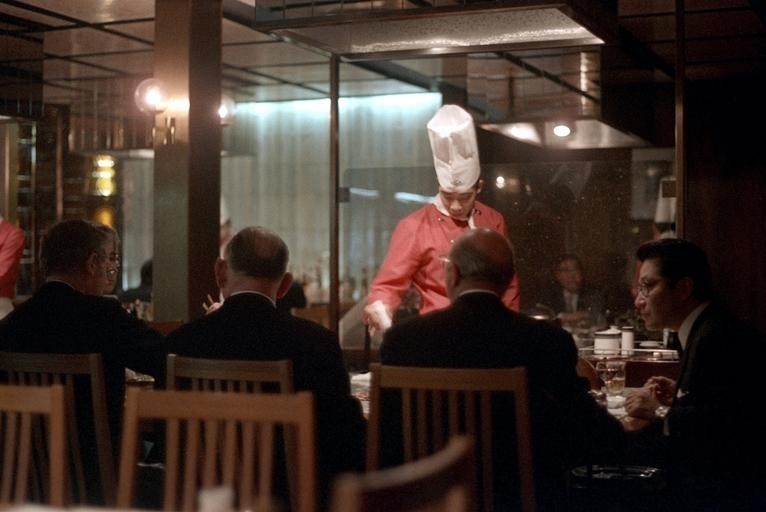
568,295,574,314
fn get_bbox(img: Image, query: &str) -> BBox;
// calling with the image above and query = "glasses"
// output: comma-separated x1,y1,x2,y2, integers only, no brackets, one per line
96,252,118,277
112,251,121,271
640,278,654,298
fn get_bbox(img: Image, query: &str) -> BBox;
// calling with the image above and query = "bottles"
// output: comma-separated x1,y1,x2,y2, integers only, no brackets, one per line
363,299,394,333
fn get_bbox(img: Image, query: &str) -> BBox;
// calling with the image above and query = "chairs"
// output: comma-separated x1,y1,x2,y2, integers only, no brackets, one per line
115,353,319,510
2,352,117,508
334,359,537,510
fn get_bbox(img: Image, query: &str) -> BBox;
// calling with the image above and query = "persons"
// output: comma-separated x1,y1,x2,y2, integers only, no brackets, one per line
376,226,629,512
616,251,663,342
0,216,165,512
623,237,766,511
0,191,371,321
357,171,522,351
533,252,617,326
162,225,367,511
504,179,577,311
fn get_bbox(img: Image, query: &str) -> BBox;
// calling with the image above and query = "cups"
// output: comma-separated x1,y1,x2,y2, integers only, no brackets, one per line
621,326,636,359
605,360,626,396
591,328,623,357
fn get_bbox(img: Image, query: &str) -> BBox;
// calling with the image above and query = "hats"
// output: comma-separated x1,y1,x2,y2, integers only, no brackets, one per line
425,104,481,194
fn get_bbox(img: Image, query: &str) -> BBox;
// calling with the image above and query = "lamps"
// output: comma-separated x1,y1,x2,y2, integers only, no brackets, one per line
135,78,177,146
